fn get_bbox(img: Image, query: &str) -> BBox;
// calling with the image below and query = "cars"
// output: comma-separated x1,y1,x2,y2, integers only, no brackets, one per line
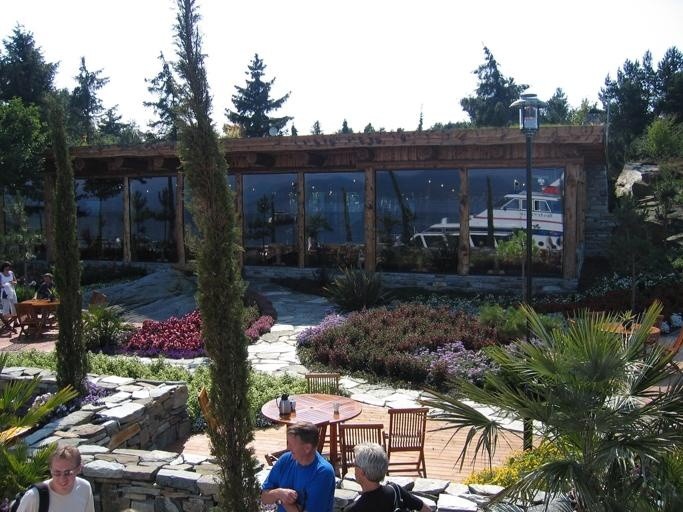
332,403,339,415
289,399,296,412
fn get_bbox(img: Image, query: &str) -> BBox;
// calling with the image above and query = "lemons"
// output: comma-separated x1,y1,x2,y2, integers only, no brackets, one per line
275,394,291,415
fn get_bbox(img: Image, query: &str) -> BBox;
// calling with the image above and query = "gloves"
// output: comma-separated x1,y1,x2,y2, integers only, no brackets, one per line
409,176,564,251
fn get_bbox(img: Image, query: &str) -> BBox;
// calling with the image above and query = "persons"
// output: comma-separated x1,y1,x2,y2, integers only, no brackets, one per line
0,262,21,327
261,421,432,512
33,273,55,300
16,445,94,512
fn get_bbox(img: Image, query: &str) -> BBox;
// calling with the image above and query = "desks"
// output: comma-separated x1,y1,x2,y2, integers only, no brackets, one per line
509,92,547,450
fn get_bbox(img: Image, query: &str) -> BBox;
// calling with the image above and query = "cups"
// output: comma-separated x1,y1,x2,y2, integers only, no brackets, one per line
51,466,78,477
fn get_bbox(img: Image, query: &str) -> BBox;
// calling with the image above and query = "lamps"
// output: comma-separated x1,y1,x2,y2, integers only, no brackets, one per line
383,406,429,479
339,423,384,481
306,372,347,449
0,291,108,341
567,309,683,377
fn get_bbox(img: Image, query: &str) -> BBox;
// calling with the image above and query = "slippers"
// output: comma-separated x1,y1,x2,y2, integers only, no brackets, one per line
9,482,50,512
386,481,417,512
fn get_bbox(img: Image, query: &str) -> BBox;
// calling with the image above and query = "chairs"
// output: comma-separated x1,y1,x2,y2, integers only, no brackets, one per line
14,322,20,327
